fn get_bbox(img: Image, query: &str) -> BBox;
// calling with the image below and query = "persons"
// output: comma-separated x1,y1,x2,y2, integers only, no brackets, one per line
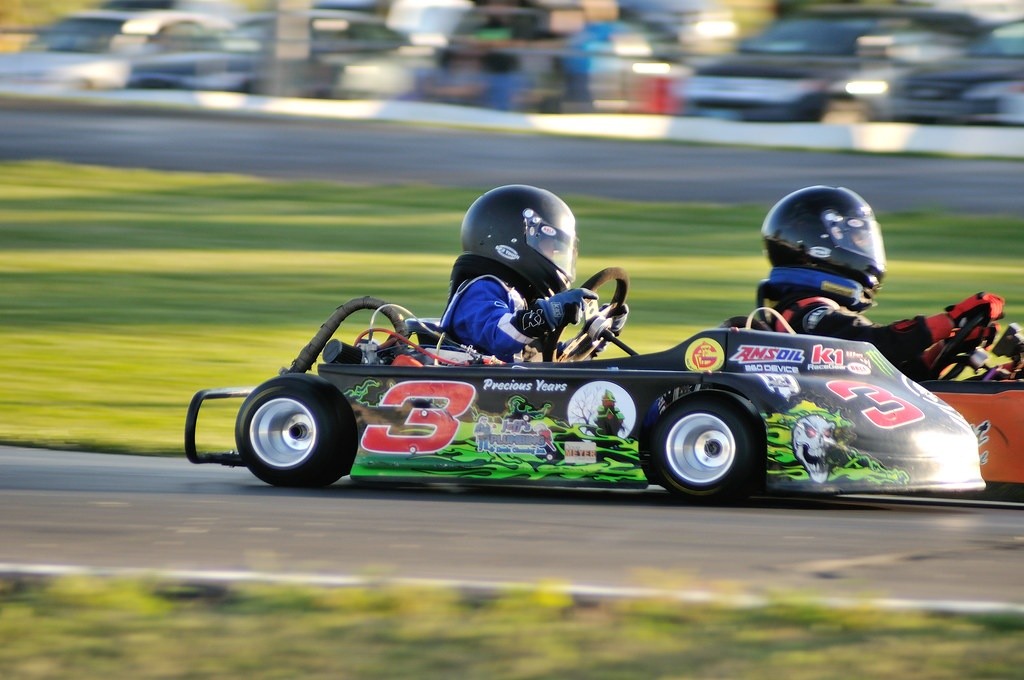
440,184,629,363
760,185,1006,382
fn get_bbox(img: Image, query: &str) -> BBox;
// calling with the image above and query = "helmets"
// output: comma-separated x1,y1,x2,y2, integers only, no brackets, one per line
461,185,578,300
760,186,887,275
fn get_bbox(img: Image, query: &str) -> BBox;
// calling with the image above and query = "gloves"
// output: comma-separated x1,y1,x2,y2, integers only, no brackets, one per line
535,288,600,332
946,289,1005,344
585,302,630,352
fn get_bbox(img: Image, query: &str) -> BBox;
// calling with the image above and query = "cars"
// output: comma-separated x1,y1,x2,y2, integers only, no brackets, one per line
1,3,1024,128
